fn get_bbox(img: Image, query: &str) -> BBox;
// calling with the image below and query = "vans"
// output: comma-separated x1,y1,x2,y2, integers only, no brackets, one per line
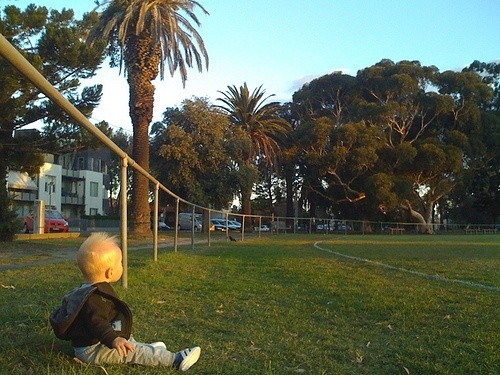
178,213,215,232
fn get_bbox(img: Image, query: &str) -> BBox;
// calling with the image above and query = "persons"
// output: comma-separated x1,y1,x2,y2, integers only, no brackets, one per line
50,233,201,371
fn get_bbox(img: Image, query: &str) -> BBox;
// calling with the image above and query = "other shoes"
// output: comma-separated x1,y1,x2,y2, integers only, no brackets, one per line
175,346,201,371
151,342,166,348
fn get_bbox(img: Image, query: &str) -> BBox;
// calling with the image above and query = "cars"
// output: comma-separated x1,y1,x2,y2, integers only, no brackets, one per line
151,221,171,231
211,219,241,231
23,209,69,233
316,222,352,232
258,225,270,232
